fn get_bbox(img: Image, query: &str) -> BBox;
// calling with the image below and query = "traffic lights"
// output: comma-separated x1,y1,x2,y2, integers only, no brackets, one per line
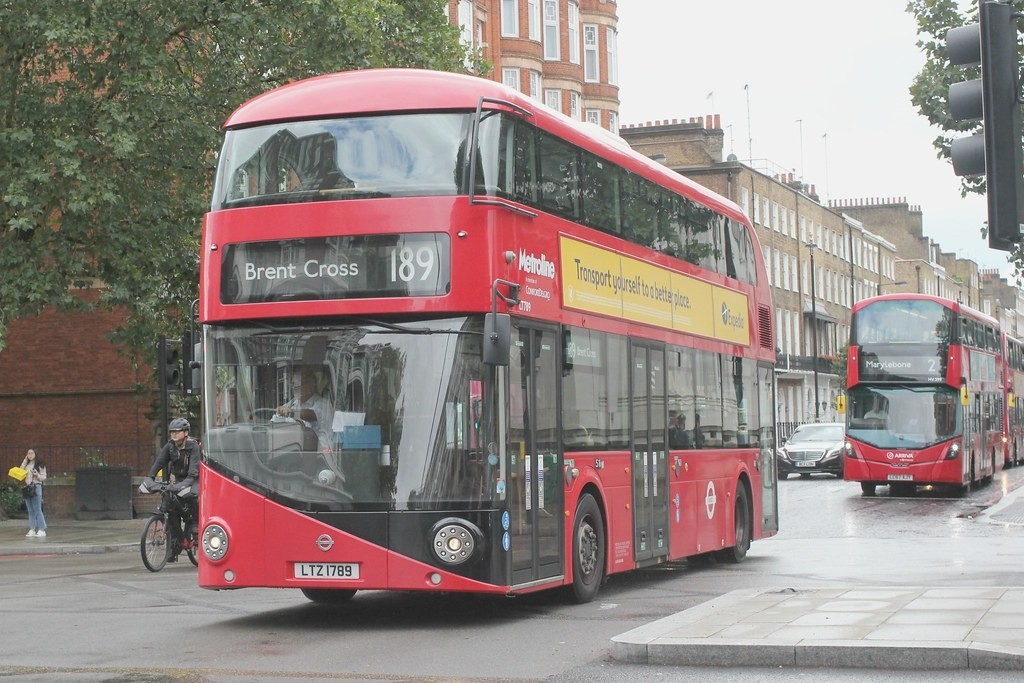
165,348,181,386
946,0,1022,253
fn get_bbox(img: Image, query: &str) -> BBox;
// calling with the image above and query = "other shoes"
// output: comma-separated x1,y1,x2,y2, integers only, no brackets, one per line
167,553,175,561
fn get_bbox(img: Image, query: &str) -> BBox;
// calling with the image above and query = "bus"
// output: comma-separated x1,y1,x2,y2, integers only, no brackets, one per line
183,68,780,605
836,292,1024,499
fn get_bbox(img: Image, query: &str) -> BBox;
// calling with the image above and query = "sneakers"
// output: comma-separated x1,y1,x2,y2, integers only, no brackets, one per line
36,530,46,537
26,529,36,537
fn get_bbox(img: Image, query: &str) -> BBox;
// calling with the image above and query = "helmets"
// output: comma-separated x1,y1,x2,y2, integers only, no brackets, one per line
169,418,190,430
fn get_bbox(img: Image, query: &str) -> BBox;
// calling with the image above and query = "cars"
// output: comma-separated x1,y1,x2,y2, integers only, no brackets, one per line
778,422,845,480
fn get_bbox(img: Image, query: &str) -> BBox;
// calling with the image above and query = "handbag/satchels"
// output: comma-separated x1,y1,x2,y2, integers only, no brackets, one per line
21,482,37,499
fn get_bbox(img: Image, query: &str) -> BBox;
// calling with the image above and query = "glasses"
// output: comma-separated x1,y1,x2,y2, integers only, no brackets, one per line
170,431,180,435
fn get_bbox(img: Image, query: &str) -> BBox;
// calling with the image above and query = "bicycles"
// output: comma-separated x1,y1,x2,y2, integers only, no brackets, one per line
140,480,199,573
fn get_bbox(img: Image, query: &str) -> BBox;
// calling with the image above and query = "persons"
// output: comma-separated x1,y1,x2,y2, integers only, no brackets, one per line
269,368,345,492
148,418,200,563
18,448,47,536
864,402,889,419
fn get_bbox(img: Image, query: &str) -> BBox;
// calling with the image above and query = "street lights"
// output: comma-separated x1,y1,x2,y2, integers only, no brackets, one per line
804,233,820,423
878,280,909,295
